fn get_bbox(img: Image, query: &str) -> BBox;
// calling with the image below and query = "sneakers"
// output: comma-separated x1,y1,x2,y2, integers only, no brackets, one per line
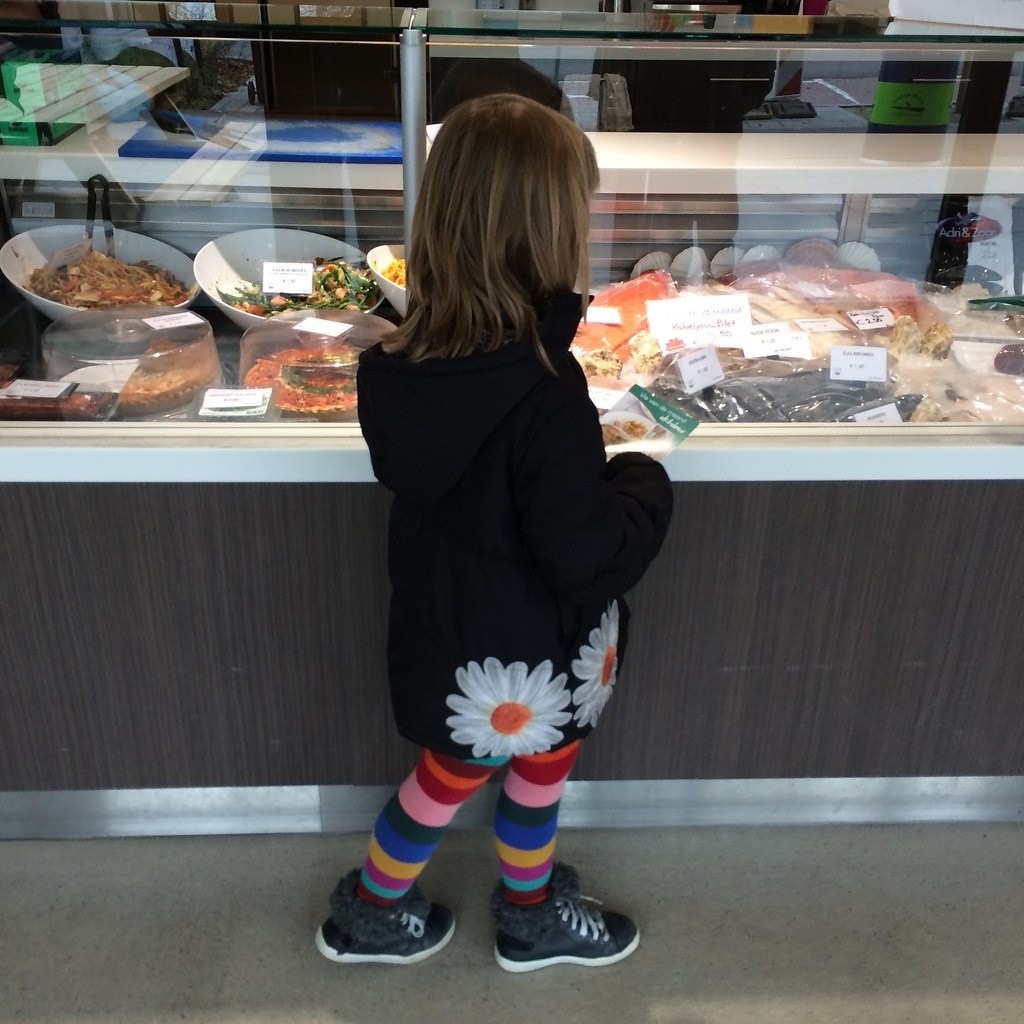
490,861,640,972
315,867,455,964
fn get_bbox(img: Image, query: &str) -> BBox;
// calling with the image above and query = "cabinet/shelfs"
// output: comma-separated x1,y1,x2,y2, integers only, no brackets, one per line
0,31,1024,837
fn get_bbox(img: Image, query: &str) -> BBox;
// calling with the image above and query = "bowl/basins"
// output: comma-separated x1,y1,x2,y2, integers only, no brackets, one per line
2,225,203,323
193,229,383,332
366,242,412,319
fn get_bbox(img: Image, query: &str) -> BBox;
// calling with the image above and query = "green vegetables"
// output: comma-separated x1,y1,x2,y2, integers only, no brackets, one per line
216,260,382,311
281,351,357,394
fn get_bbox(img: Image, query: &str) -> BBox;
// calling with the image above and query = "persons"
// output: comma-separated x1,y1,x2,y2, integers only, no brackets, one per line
313,93,672,972
607,0,775,292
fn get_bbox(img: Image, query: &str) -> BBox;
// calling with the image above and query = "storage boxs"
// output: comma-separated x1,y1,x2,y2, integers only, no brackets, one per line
55,0,427,29
712,15,816,35
